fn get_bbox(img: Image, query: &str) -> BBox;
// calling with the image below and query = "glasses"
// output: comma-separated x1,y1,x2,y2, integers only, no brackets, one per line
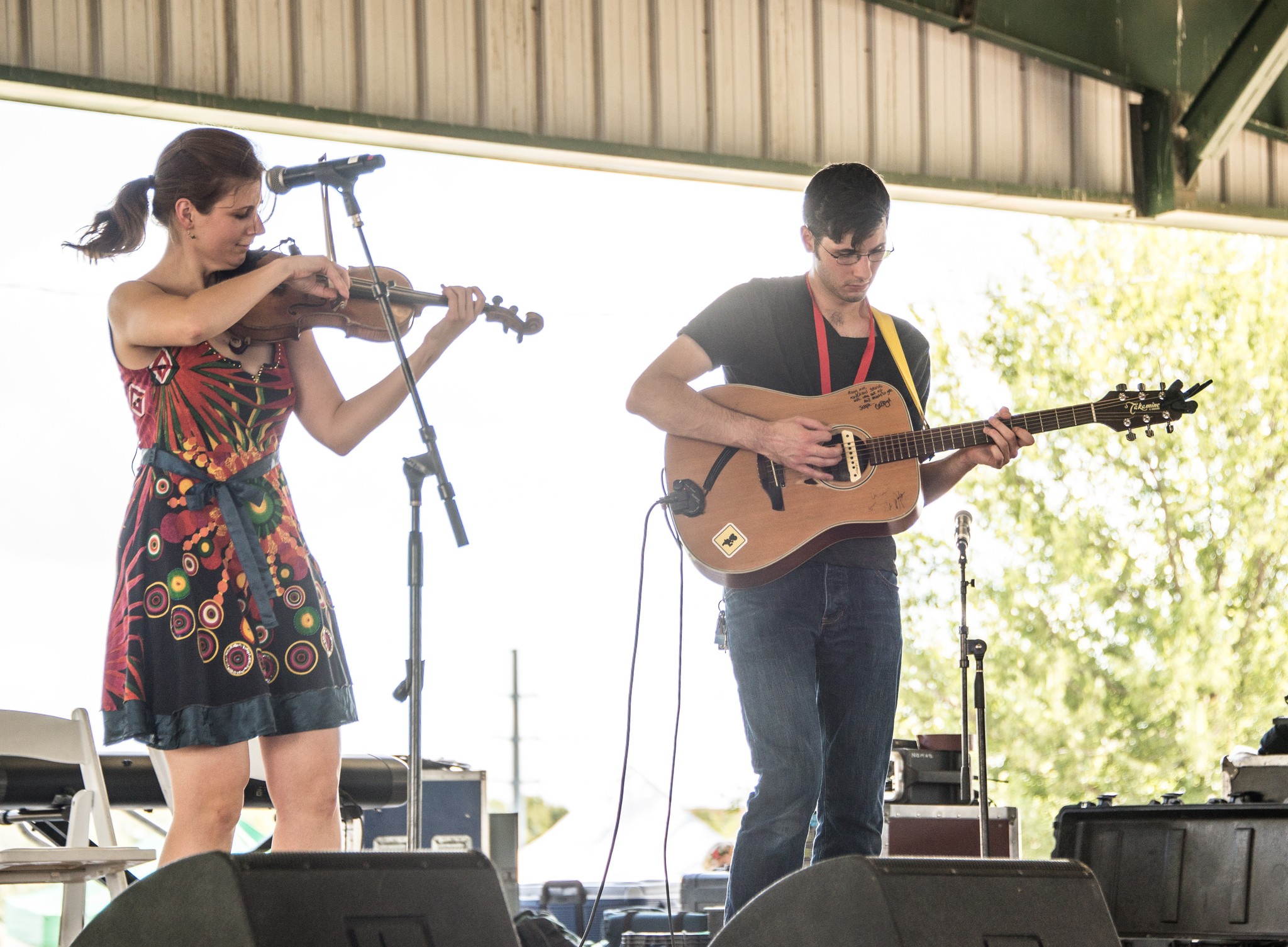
805,223,895,264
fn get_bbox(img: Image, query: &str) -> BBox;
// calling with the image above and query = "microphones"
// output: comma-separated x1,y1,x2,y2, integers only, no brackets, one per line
954,510,972,551
265,153,385,195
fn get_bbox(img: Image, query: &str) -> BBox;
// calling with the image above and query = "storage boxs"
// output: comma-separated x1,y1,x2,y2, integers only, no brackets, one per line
881,804,1021,860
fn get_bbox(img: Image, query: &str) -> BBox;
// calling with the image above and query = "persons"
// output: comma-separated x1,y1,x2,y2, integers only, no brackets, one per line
58,128,487,871
627,162,1034,928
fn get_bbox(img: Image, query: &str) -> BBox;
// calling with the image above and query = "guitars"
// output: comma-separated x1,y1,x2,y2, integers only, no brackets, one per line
662,375,1218,590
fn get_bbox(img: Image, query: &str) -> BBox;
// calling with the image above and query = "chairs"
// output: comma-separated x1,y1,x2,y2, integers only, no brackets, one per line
0,708,156,947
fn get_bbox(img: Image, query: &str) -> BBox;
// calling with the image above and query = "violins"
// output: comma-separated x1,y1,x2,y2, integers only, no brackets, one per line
203,249,544,347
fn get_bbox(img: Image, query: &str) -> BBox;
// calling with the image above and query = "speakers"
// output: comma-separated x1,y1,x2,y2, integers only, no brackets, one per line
68,849,522,947
706,801,1288,947
